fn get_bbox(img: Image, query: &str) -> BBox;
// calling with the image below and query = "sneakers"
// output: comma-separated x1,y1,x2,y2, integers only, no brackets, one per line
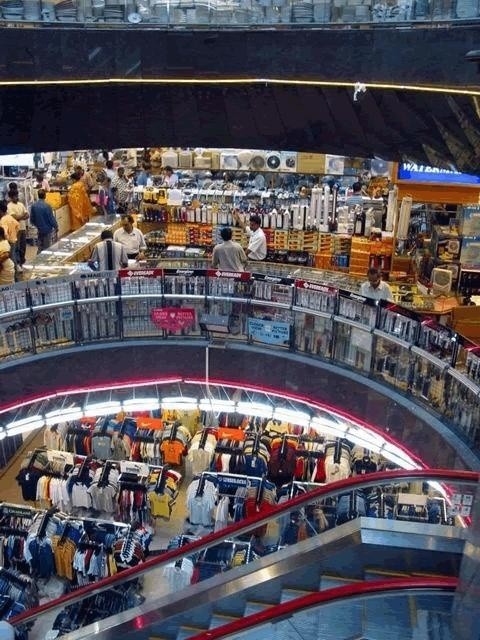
16,264,23,273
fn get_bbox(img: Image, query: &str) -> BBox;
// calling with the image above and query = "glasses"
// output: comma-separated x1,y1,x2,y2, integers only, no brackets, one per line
368,276,378,282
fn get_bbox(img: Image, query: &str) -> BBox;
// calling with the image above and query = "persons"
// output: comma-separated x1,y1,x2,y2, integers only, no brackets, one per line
0,147,181,286
425,497,440,525
211,228,248,272
345,182,373,210
231,209,268,262
358,268,394,301
312,508,329,538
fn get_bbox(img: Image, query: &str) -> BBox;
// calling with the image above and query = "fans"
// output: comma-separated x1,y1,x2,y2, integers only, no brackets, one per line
220,152,297,190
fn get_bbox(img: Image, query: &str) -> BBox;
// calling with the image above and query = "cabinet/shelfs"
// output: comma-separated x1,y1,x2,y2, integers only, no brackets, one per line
22,216,368,294
